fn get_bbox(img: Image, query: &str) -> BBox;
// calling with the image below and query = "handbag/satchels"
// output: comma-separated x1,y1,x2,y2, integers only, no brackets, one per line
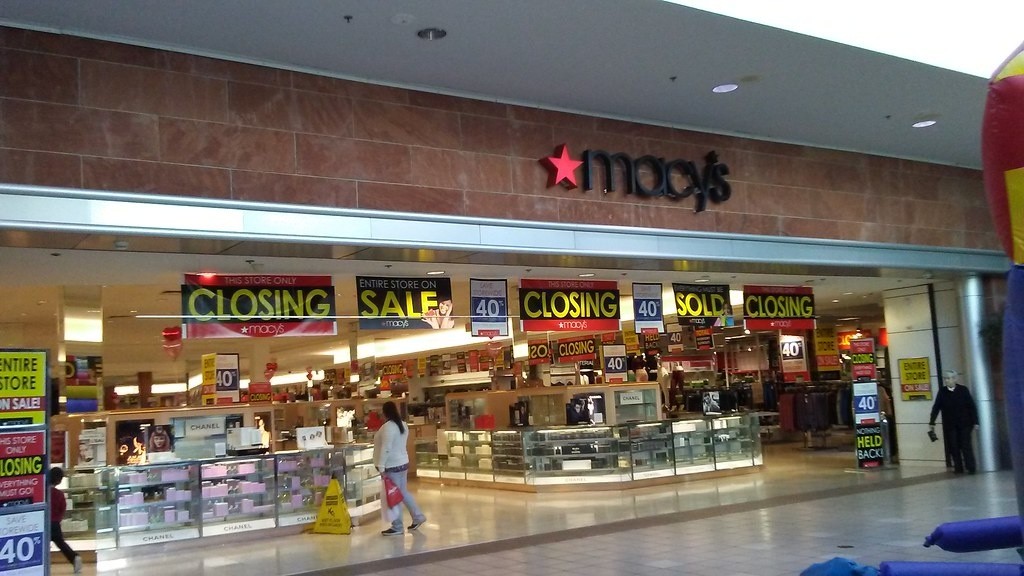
384,477,404,509
927,430,938,442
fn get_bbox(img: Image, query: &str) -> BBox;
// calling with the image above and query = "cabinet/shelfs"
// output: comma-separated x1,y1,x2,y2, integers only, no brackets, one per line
45,396,761,552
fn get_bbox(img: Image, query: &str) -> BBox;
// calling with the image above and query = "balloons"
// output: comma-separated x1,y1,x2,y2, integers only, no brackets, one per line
163,340,183,359
264,363,278,381
162,326,182,344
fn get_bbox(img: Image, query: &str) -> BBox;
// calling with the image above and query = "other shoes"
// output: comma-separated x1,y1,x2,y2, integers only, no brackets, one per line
407,516,426,530
967,467,976,474
953,467,963,473
74,555,82,574
381,528,404,535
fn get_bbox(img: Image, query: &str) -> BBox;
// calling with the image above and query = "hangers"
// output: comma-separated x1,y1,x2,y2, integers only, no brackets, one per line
785,384,848,394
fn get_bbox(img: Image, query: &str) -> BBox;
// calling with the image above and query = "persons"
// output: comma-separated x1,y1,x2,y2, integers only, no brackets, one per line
714,301,731,326
421,297,454,328
373,402,426,536
636,369,648,382
259,419,269,447
670,366,686,412
658,366,670,407
49,467,82,573
703,393,720,411
928,369,980,475
78,443,94,465
150,430,170,452
